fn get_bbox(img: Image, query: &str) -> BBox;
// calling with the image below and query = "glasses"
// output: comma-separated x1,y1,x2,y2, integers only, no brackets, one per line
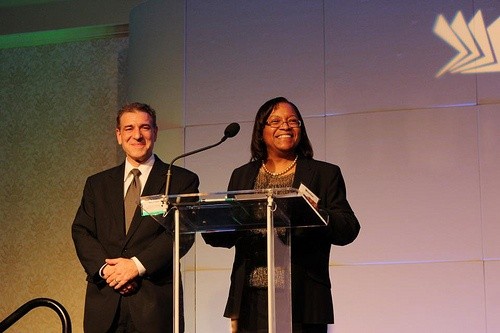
262,119,301,128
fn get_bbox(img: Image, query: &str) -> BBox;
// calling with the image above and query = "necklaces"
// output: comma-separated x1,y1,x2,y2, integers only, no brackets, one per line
262,155,298,176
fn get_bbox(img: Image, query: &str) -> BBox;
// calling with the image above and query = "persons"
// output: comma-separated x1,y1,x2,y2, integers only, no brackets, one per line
201,97,361,333
72,102,199,333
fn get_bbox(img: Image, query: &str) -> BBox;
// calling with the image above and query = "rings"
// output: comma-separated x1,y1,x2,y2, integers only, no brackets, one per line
115,279,119,284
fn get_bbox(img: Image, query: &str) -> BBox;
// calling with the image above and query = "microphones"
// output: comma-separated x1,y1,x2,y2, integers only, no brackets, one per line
168,122,240,173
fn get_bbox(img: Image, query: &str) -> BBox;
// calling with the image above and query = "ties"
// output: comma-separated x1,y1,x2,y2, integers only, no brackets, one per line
124,169,141,236
